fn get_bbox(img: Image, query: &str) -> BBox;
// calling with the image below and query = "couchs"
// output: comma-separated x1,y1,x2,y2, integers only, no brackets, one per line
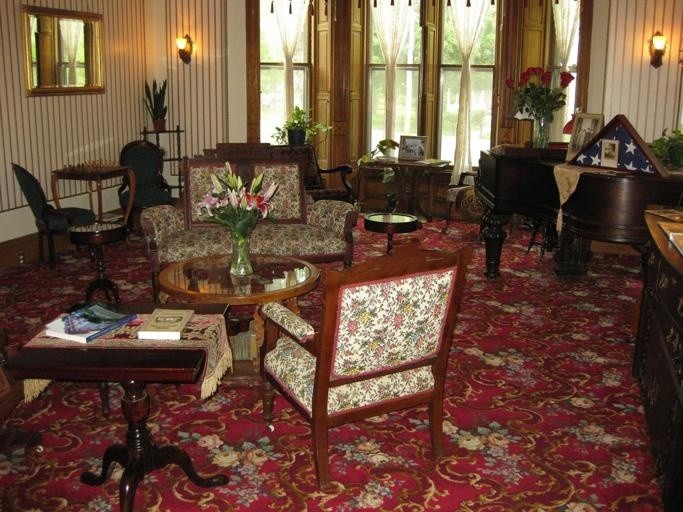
139,156,359,268
202,142,353,203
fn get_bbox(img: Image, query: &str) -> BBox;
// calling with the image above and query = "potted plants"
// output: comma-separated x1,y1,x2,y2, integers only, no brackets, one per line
270,106,335,146
142,78,168,131
377,139,399,158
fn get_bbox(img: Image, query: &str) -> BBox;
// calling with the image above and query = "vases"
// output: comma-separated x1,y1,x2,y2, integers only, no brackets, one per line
229,231,254,277
532,118,550,148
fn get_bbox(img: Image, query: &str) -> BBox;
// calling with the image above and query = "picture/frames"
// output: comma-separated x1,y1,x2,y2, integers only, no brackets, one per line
600,139,619,168
565,113,604,163
397,135,429,161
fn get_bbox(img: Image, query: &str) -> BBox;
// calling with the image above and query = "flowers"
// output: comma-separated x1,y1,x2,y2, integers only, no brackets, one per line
195,160,279,271
505,66,575,141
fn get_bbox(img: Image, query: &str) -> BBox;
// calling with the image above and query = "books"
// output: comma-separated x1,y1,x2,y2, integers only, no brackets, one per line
44,303,136,344
134,307,195,342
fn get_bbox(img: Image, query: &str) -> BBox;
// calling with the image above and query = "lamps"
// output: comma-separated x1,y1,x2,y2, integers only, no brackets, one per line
648,30,668,69
175,34,194,64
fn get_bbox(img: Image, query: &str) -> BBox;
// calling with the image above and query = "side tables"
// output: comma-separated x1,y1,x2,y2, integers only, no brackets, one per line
52,163,136,227
376,159,450,223
7,302,231,512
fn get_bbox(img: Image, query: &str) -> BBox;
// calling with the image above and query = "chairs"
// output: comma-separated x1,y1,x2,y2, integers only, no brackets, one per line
11,163,96,262
440,171,479,234
257,241,475,490
117,139,172,228
